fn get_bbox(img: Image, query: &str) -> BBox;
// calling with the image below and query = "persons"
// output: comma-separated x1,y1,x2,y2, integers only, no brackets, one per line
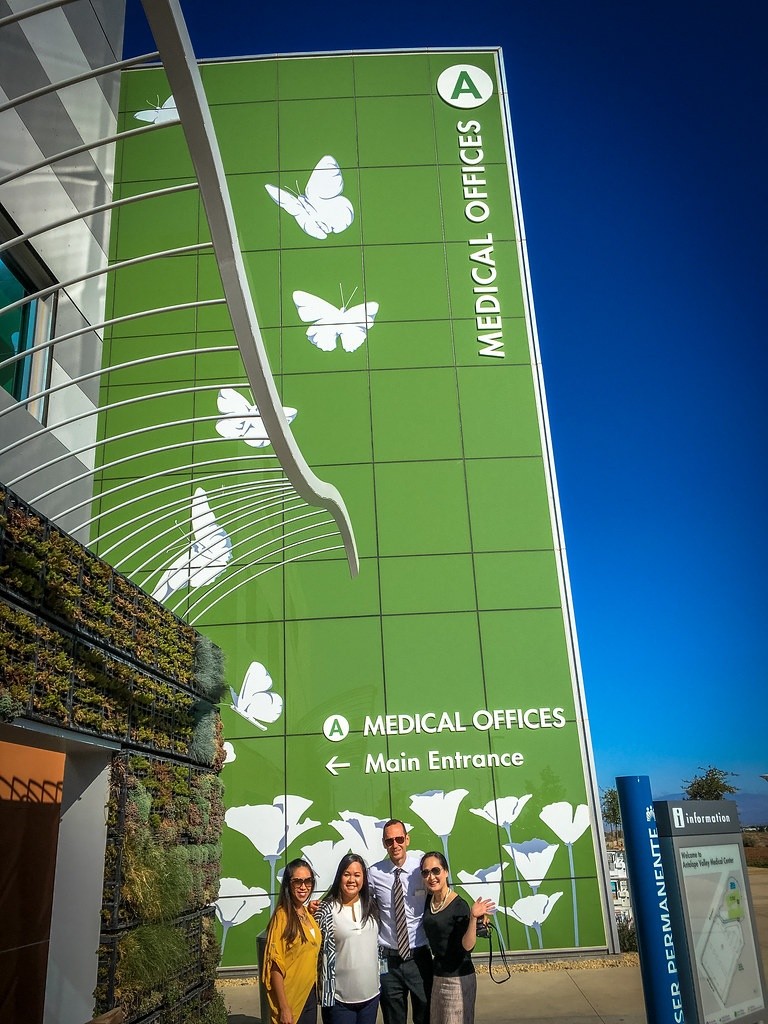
313,854,381,1024
308,819,490,1024
420,851,495,1024
262,858,322,1024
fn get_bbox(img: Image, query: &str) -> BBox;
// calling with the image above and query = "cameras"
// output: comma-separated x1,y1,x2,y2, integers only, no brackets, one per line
475,917,488,938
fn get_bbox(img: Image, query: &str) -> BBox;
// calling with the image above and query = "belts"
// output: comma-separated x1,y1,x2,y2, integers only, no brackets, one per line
380,945,428,957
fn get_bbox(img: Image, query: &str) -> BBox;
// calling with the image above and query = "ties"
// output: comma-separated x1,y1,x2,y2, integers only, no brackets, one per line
393,868,410,961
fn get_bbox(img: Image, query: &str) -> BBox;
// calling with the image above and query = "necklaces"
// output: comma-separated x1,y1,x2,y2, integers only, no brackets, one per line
431,887,451,914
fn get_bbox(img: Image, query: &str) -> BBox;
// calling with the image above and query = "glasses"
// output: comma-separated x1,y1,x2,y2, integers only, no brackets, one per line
384,836,407,847
290,877,315,889
421,867,445,878
